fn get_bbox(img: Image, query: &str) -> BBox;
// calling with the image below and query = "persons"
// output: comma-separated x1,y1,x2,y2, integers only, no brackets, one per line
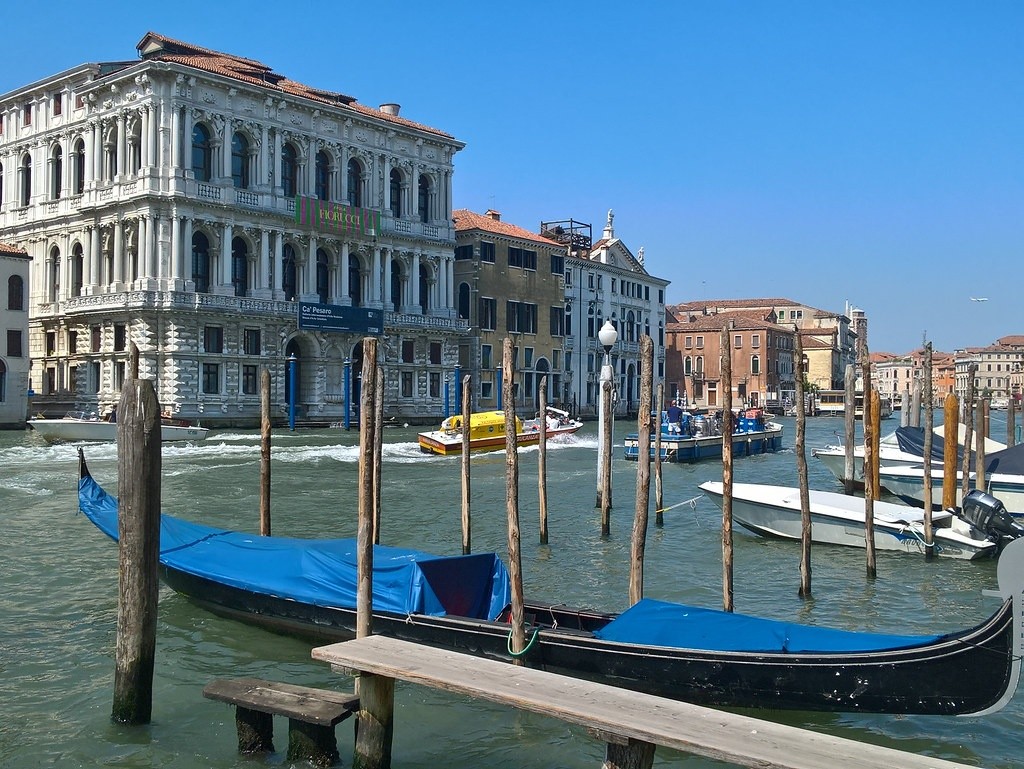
667,399,682,436
108,405,117,423
546,412,560,430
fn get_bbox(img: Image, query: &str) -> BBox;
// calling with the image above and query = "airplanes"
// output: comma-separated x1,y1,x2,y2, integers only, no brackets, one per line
970,295,990,304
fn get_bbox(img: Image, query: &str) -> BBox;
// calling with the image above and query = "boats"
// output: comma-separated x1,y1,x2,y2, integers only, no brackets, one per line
76,444,1024,718
855,395,893,420
27,413,210,446
810,421,1023,515
416,405,585,457
624,407,783,464
696,479,1023,560
